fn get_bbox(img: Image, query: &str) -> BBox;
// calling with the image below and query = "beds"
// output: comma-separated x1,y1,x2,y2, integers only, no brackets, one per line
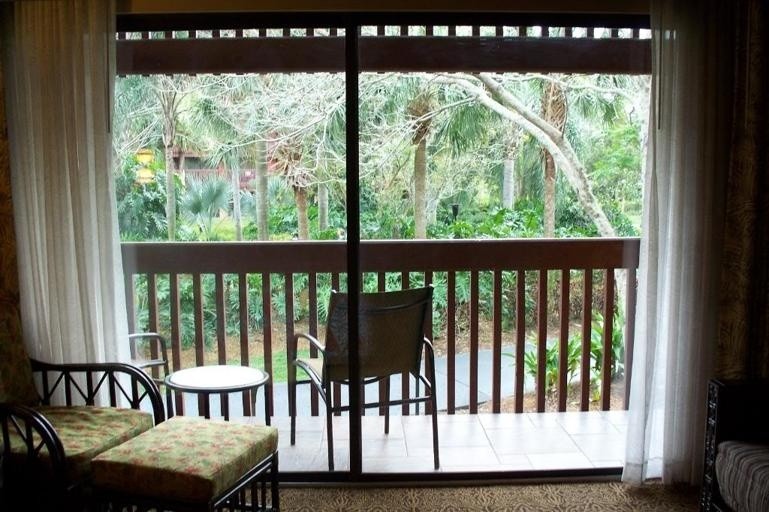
699,376,769,512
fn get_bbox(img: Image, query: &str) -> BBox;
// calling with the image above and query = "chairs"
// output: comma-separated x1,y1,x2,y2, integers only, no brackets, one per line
129,332,173,419
0,299,165,511
287,285,440,470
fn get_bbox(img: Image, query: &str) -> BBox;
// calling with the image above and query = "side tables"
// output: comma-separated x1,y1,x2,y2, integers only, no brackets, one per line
165,364,271,427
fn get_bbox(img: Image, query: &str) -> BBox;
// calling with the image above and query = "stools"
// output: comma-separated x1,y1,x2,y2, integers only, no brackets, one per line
90,415,280,512
